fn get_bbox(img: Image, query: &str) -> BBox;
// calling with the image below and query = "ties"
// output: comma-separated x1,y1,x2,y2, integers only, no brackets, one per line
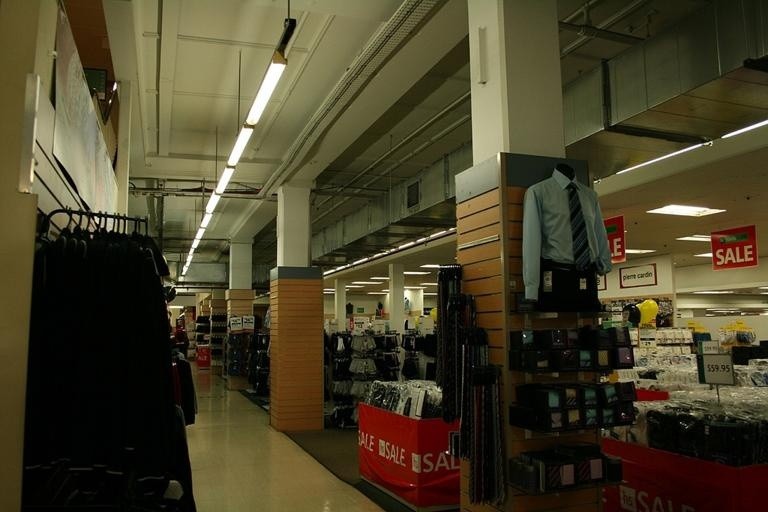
567,183,591,271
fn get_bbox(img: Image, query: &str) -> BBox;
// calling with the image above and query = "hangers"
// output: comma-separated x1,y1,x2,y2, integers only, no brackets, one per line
35,206,158,275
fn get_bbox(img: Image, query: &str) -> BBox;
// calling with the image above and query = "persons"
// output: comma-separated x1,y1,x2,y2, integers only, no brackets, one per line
173,326,189,359
523,163,613,301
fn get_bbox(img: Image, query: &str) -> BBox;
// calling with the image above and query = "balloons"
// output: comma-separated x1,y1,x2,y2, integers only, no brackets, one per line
430,308,437,322
623,300,658,327
